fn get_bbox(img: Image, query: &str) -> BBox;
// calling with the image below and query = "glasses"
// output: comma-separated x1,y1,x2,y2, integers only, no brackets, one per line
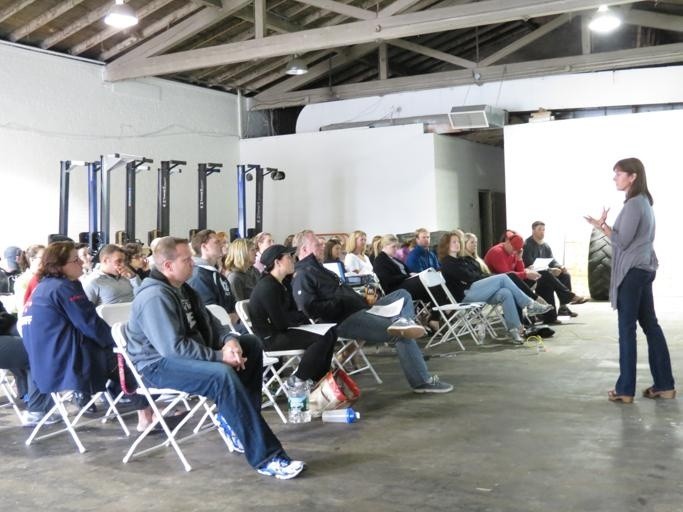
66,258,80,264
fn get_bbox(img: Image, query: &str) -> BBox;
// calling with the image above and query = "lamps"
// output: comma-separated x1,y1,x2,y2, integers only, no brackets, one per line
588,4,621,34
103,0,139,29
284,54,308,76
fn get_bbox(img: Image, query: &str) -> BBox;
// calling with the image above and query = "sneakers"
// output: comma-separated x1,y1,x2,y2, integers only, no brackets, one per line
214,413,246,454
413,375,453,393
386,322,425,338
21,410,62,426
256,456,307,479
505,296,589,344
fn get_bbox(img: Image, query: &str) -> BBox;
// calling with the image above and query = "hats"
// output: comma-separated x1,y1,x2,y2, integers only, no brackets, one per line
260,245,296,266
5,247,22,268
505,231,522,250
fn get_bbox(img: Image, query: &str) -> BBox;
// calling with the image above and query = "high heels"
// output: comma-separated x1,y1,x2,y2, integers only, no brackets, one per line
643,387,675,399
609,391,632,403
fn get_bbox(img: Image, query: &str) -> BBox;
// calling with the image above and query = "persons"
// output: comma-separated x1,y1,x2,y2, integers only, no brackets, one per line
291,229,456,392
249,244,338,389
582,158,677,406
1,229,301,429
319,221,589,343
125,237,309,479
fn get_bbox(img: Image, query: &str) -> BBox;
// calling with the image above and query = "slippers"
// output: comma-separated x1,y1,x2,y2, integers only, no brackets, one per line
154,410,190,430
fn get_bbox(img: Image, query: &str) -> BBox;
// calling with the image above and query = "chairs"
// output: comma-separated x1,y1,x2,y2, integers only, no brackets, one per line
111,323,233,472
235,298,306,407
0,368,26,425
191,303,288,433
17,304,129,454
0,295,67,398
412,271,542,351
226,272,241,301
308,317,383,385
94,302,196,424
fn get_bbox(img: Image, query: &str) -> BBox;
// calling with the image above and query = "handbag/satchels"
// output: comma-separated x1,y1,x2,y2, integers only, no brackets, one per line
309,368,360,419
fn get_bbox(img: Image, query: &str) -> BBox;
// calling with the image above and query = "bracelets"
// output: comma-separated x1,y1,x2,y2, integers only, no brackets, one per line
600,223,607,231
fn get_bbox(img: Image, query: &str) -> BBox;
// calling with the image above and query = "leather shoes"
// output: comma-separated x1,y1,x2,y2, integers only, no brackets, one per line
74,391,96,414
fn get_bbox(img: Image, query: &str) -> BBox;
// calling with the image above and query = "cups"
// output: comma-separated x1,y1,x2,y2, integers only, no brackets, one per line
367,284,375,294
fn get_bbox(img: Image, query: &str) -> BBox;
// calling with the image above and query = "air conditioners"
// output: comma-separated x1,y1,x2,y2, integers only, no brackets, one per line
449,105,506,130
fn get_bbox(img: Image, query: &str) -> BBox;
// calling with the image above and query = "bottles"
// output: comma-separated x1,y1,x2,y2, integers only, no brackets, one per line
287,382,311,424
478,324,486,345
322,408,360,424
